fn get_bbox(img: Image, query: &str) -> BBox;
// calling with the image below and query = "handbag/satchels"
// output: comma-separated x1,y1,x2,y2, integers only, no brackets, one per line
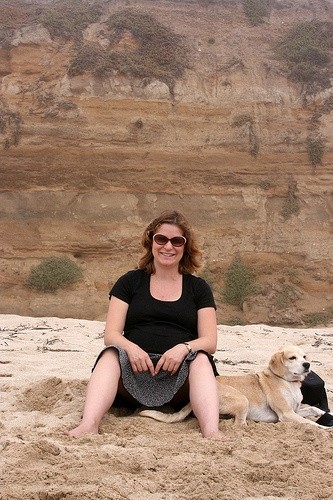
300,370,330,412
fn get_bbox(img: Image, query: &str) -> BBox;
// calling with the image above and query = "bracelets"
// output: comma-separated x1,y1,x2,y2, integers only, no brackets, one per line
179,342,192,353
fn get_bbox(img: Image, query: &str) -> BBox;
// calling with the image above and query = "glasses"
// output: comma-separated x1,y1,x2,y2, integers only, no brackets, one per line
153,233,186,247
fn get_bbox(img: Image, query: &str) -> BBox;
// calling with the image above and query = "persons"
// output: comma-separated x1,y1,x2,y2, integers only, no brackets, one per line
70,211,220,438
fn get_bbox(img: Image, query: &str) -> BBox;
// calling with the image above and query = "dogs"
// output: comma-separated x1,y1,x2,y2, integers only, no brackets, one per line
138,346,332,430
